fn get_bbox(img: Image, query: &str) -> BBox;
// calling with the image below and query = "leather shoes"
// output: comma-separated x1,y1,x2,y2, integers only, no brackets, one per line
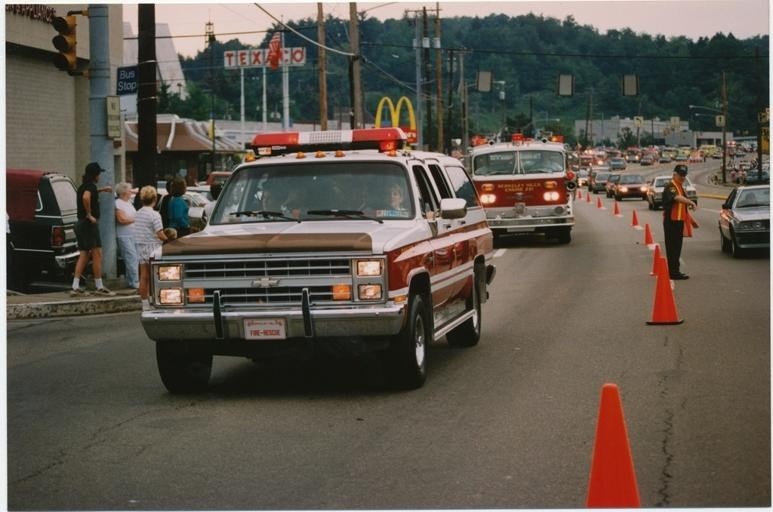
670,273,689,280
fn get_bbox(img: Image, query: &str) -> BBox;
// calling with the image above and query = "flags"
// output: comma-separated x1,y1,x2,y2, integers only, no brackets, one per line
266,25,282,70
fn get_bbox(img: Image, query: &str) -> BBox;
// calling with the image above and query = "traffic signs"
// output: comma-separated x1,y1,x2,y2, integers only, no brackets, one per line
569,141,769,193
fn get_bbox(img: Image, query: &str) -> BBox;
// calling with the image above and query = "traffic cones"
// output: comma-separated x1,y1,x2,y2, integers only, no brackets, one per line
613,200,621,215
632,209,640,227
643,257,684,327
578,189,583,199
648,245,664,276
597,196,603,208
586,381,645,507
644,224,654,245
586,192,591,202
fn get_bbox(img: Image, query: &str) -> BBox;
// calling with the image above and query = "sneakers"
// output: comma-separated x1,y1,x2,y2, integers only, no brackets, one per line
70,289,90,297
94,288,116,297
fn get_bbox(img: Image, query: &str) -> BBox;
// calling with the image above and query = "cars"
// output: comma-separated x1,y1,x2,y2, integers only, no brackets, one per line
647,175,698,209
591,172,612,194
604,174,621,198
716,183,770,257
120,177,263,243
614,174,648,201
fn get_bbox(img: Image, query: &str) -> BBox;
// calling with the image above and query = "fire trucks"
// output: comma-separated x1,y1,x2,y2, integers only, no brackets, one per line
466,133,576,248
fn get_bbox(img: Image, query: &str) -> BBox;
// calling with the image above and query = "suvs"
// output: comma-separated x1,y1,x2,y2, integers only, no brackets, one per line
6,167,91,287
137,125,498,392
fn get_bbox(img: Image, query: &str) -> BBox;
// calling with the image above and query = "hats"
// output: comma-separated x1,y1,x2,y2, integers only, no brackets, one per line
85,163,105,173
675,165,687,175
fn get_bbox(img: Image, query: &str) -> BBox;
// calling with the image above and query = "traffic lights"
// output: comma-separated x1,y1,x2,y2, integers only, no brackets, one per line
49,14,77,75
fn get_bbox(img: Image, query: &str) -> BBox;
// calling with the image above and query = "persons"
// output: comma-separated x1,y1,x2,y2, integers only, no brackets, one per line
162,228,178,242
373,179,406,214
156,175,192,240
662,165,698,280
136,186,174,312
473,154,496,175
200,181,237,225
69,162,113,295
258,180,289,215
746,193,757,205
529,152,562,172
112,180,140,289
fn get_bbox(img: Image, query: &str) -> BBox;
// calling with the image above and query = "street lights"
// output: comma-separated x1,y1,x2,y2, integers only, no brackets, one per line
519,117,560,132
760,127,771,153
465,79,507,145
694,113,716,118
688,104,730,116
207,35,218,174
